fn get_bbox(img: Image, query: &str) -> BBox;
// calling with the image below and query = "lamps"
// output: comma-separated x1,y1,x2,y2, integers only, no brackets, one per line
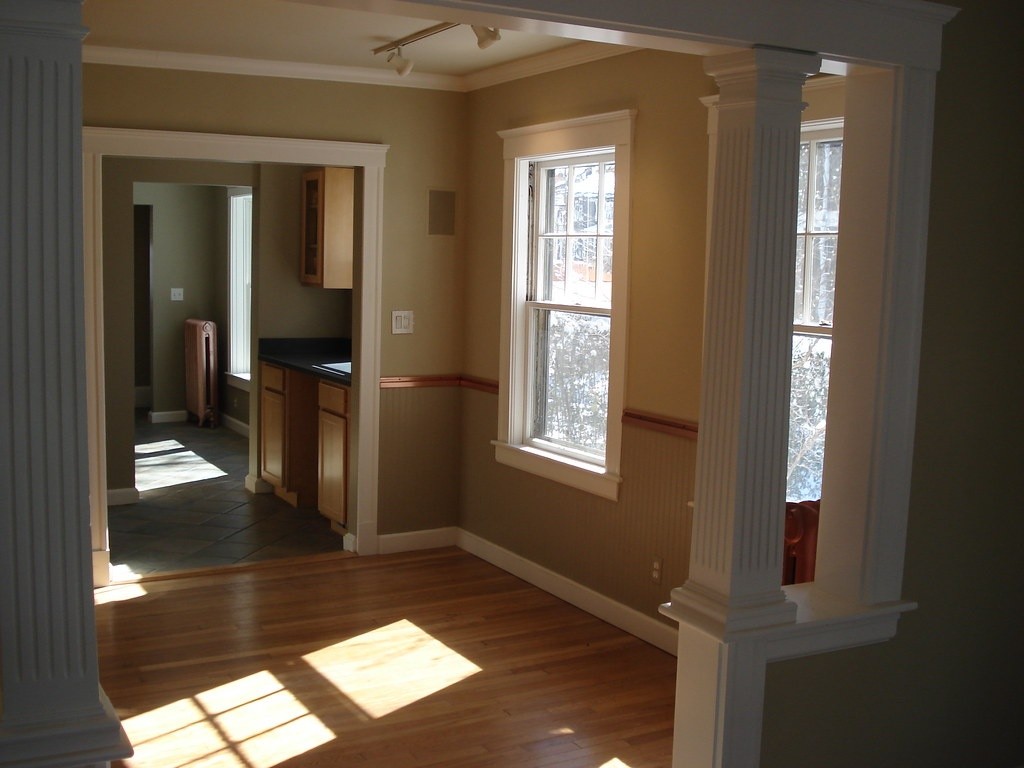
370,23,501,81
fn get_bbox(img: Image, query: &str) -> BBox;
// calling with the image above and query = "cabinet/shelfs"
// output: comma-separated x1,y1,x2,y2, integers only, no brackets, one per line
300,166,353,289
255,361,351,536
